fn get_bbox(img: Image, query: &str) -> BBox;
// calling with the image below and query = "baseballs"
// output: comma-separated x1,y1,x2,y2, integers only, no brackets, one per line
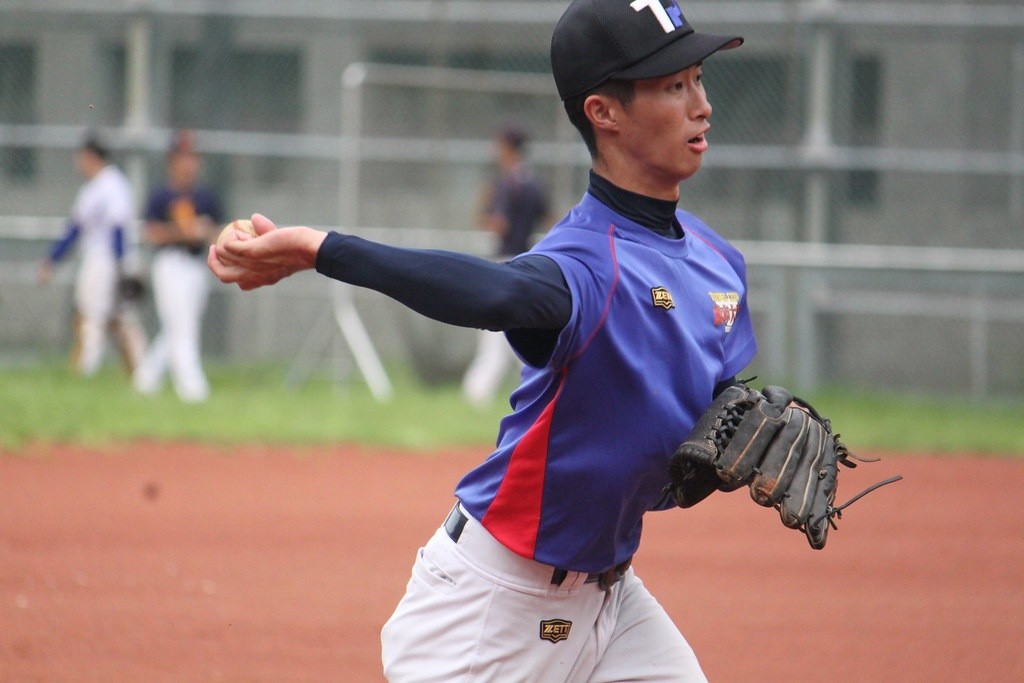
210,215,267,265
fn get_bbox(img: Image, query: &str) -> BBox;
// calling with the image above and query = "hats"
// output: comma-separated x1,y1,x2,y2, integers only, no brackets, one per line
551,0,744,101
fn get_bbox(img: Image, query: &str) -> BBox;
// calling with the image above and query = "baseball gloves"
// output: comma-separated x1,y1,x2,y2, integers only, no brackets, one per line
654,374,903,552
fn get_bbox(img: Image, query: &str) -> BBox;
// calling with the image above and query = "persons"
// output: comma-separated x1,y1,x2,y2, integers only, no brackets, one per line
40,126,228,405
461,132,550,412
209,1,905,681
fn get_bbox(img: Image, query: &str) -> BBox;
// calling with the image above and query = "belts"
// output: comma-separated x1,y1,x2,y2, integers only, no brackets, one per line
444,501,633,590
